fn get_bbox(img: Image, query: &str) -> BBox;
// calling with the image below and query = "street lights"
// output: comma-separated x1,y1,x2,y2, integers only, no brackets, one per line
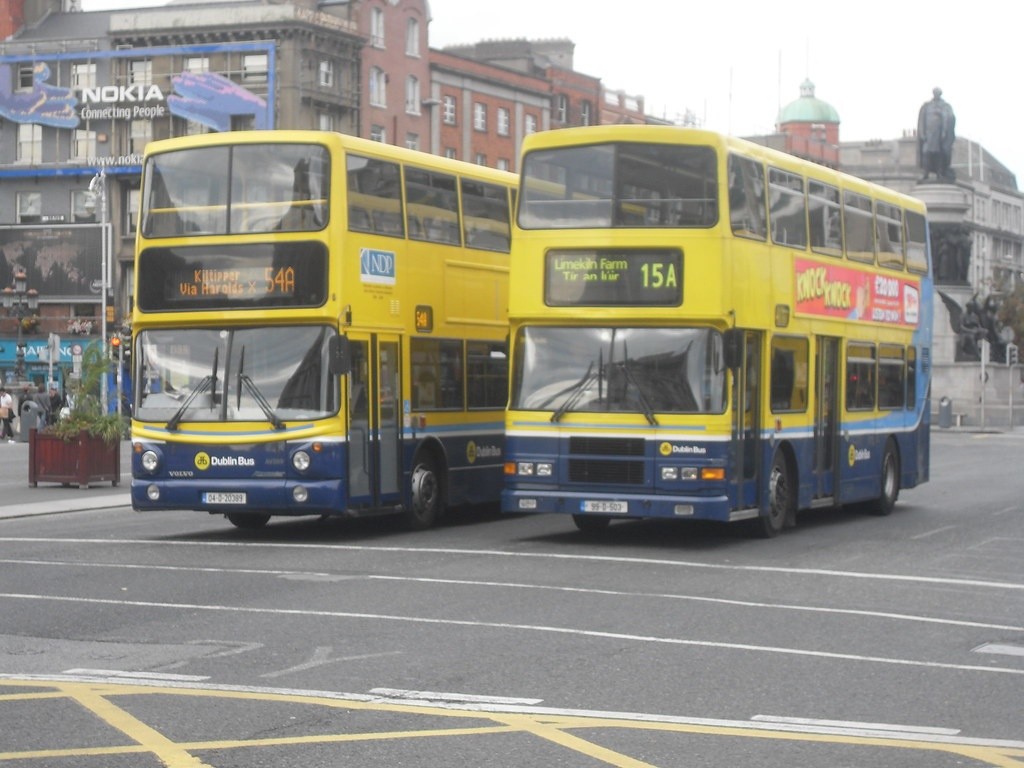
0,270,39,388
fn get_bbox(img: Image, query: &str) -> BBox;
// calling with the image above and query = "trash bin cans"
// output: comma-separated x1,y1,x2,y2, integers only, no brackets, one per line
20,400,38,442
938,396,951,428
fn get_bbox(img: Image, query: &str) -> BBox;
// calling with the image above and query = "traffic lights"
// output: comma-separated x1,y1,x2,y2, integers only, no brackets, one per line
1009,347,1016,365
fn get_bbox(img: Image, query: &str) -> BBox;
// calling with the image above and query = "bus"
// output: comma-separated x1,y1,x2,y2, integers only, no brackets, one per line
501,125,934,539
128,130,647,530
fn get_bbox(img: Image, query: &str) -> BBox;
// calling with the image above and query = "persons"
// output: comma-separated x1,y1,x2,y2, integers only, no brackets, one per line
915,88,956,179
18,384,33,417
938,236,952,279
32,383,51,433
0,387,17,443
958,300,994,361
48,386,63,425
